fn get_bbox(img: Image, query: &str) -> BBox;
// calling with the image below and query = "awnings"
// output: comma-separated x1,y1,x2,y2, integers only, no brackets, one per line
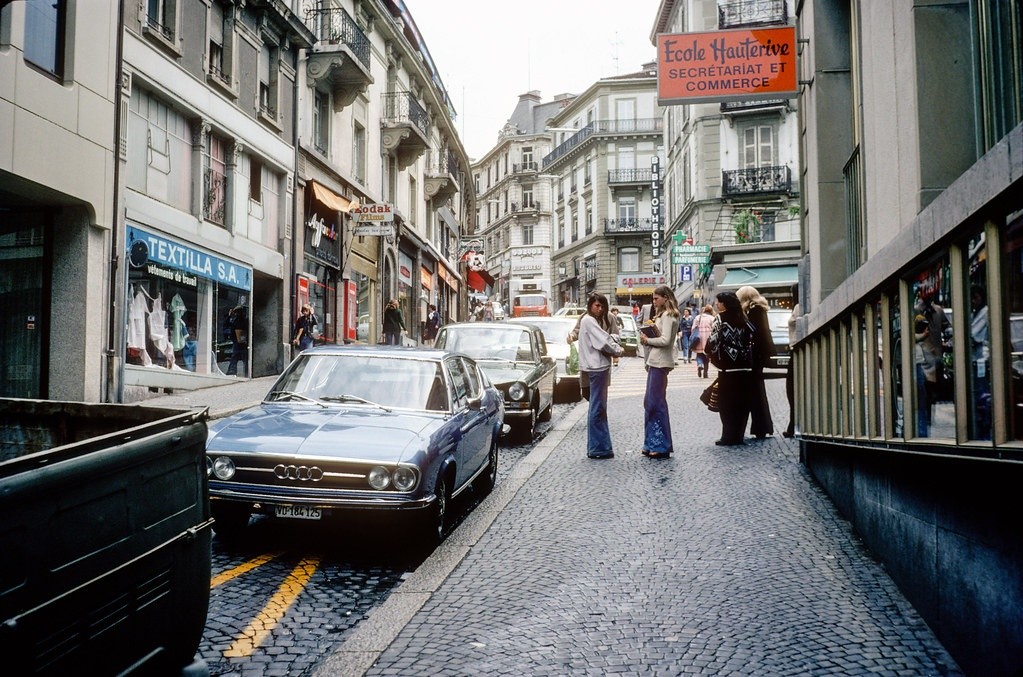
615,286,656,296
476,264,495,288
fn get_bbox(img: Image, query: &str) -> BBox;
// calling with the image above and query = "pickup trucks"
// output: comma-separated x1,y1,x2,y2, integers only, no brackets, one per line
0,393,215,677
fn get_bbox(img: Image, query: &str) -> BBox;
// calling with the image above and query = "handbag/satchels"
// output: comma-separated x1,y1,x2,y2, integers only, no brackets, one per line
423,330,430,340
699,377,720,412
688,327,701,350
307,314,317,339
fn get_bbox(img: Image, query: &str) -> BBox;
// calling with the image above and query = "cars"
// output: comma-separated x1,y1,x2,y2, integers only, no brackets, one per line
763,309,792,368
431,319,559,445
499,306,587,403
465,291,507,321
202,343,510,556
617,313,641,358
942,307,1023,441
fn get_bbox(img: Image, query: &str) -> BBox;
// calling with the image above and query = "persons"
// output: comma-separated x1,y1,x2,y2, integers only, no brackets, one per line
471,299,494,321
226,298,248,376
610,308,624,366
423,304,439,349
567,290,619,402
680,306,694,363
912,284,952,438
736,285,777,440
641,286,680,457
633,302,638,321
382,299,408,346
578,295,624,459
691,303,716,377
564,299,577,308
704,291,756,446
637,293,658,371
782,282,799,438
293,303,318,365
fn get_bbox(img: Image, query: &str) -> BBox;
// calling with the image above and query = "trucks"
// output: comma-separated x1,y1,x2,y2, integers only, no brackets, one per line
512,293,548,318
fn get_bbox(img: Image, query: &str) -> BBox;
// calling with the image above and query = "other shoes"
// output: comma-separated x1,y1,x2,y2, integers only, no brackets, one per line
703,373,708,378
587,450,614,460
756,425,774,438
642,448,670,458
782,431,795,437
683,358,687,364
715,437,744,446
697,367,702,377
